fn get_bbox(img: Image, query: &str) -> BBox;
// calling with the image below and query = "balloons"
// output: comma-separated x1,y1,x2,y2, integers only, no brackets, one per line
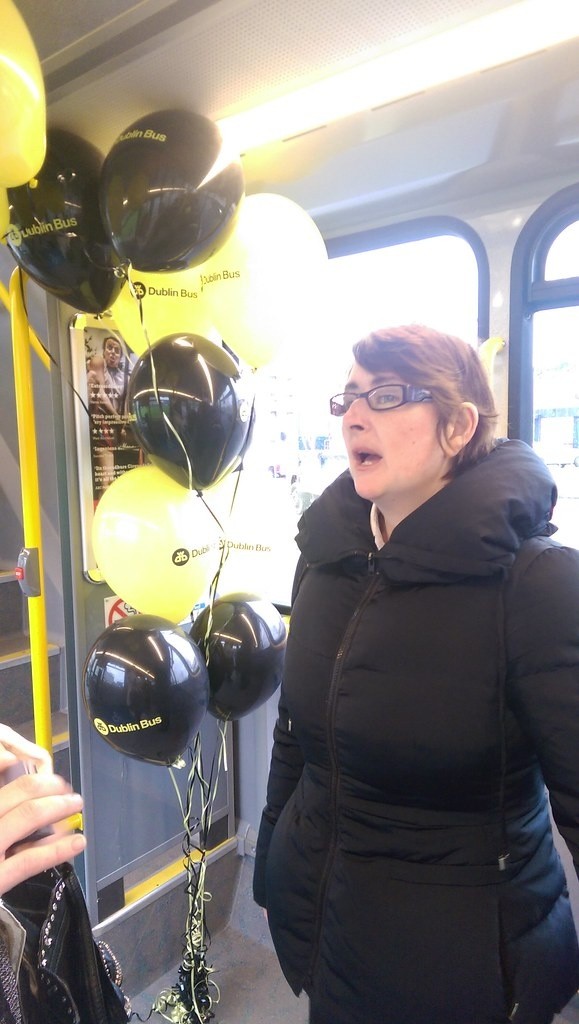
187,592,288,722
91,465,231,626
81,613,211,769
0,0,335,494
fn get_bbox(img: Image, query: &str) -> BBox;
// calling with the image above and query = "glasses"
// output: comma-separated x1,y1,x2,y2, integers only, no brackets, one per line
331,383,435,416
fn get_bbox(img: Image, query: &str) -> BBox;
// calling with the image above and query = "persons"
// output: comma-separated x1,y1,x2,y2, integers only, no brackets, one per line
0,722,88,899
252,323,579,1024
89,335,135,499
